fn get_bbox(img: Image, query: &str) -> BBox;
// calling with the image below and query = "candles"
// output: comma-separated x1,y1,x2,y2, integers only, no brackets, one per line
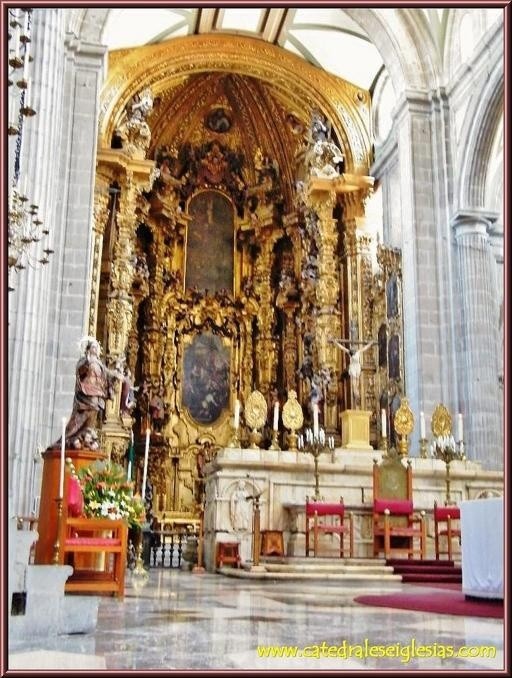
381,408,388,436
456,414,464,442
313,405,321,441
233,399,240,431
273,401,280,432
419,412,427,438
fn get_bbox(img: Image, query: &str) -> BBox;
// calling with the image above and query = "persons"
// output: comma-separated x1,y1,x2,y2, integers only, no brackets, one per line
190,352,225,418
312,119,327,144
332,341,374,400
63,336,140,449
132,99,151,122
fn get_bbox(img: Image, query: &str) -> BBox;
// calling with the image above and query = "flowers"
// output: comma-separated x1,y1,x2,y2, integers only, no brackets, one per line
73,460,147,528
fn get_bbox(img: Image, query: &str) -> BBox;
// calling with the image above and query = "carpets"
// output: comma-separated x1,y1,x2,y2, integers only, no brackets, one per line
353,582,504,619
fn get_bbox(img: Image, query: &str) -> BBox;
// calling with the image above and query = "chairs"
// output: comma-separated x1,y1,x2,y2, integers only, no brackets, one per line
374,498,461,560
305,494,356,558
58,457,128,602
